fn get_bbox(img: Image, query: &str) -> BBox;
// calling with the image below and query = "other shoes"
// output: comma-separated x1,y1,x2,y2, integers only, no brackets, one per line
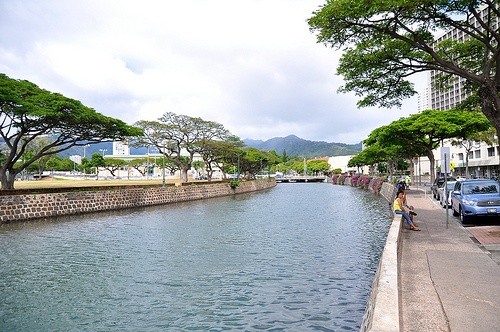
414,223,418,227
409,211,417,216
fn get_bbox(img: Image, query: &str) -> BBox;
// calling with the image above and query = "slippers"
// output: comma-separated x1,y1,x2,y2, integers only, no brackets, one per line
414,228,420,231
410,227,414,230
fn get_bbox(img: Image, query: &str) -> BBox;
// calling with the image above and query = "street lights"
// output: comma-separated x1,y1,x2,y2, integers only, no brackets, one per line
84,144,90,157
100,148,107,157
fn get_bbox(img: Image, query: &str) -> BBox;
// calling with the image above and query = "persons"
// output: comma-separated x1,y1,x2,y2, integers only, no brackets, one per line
396,178,406,190
398,187,418,227
394,191,421,231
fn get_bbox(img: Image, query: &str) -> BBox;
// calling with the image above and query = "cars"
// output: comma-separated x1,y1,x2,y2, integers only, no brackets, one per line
433,176,466,207
451,179,500,224
